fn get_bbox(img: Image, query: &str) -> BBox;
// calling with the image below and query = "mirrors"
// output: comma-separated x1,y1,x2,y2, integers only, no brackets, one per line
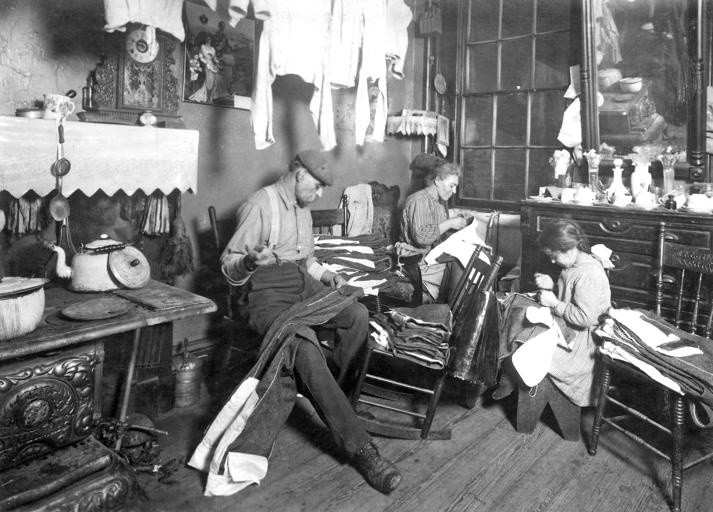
579,0,708,170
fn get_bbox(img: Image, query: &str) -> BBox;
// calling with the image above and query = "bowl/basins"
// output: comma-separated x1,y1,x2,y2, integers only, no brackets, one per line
620,77,643,93
598,68,622,91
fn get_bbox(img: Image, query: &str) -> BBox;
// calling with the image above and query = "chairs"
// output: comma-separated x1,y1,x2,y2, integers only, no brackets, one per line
351,242,507,440
589,221,713,510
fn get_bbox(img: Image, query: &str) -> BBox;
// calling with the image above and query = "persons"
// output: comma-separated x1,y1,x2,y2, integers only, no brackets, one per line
219,149,404,494
490,217,613,407
396,161,469,296
187,21,249,105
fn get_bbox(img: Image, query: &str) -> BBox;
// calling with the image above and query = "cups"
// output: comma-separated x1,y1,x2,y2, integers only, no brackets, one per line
42,93,74,119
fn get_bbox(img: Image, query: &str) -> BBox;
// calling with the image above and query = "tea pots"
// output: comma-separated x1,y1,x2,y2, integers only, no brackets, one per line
51,234,151,293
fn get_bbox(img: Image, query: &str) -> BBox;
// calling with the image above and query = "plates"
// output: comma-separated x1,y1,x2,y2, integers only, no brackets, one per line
61,297,131,322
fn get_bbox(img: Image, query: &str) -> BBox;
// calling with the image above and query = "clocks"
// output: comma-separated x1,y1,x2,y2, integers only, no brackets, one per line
76,22,187,128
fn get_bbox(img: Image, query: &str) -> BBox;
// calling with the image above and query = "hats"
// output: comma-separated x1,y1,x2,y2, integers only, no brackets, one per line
299,151,333,185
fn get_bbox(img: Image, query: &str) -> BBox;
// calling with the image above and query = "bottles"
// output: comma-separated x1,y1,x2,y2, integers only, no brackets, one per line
605,159,633,205
664,195,677,211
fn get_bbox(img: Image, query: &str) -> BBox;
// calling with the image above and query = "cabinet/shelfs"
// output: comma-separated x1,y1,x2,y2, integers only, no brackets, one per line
599,77,670,155
518,201,713,347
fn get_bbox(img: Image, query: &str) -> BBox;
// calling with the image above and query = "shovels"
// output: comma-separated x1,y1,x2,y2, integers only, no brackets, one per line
49,124,71,221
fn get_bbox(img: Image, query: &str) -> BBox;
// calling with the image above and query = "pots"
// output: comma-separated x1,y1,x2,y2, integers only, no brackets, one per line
1,276,48,341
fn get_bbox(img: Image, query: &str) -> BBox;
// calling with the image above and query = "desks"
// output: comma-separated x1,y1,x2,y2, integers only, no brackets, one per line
0,279,221,511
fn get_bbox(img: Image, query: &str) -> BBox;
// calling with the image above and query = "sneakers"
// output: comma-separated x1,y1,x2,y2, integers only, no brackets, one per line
353,443,402,493
493,365,517,400
